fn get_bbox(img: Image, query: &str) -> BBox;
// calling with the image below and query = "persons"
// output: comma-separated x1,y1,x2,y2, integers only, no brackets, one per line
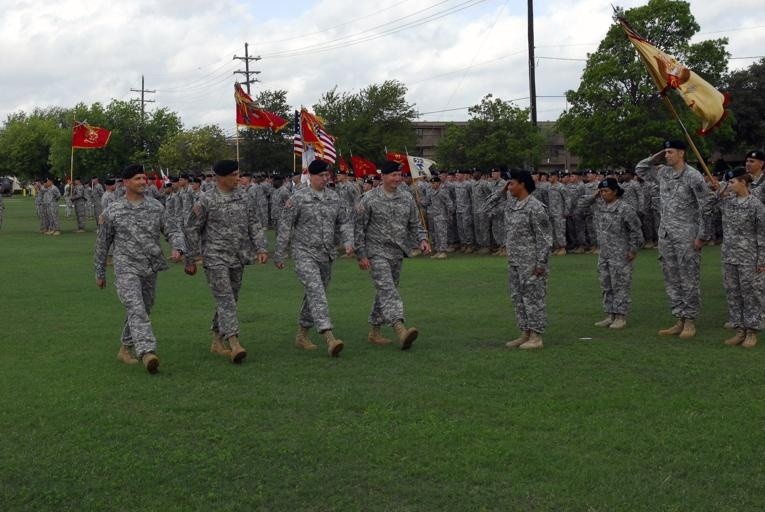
35,177,126,258
502,169,553,350
608,168,662,249
634,137,715,338
530,169,604,256
710,149,765,330
148,173,218,230
352,160,432,350
184,159,269,364
93,164,188,374
591,177,647,328
272,159,356,357
239,171,305,256
701,167,727,245
328,171,382,253
402,172,511,260
720,166,765,348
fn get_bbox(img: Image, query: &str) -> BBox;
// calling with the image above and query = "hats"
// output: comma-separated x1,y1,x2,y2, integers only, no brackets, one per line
33,167,750,189
212,160,238,176
307,159,331,175
120,163,145,180
746,150,765,161
663,140,687,151
380,160,402,174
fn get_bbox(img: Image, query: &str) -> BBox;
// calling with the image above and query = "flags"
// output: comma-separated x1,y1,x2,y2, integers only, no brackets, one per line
408,155,437,180
161,169,170,185
293,107,436,186
233,82,290,134
70,120,112,149
616,16,731,137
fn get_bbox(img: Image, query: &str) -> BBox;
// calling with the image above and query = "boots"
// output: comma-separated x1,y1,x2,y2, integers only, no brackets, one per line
394,321,418,350
116,343,139,365
658,318,697,339
294,326,318,351
142,351,159,374
367,327,393,346
227,335,246,364
409,238,663,260
38,228,98,237
723,319,759,349
593,314,627,330
321,329,344,356
504,330,545,350
210,330,232,356
